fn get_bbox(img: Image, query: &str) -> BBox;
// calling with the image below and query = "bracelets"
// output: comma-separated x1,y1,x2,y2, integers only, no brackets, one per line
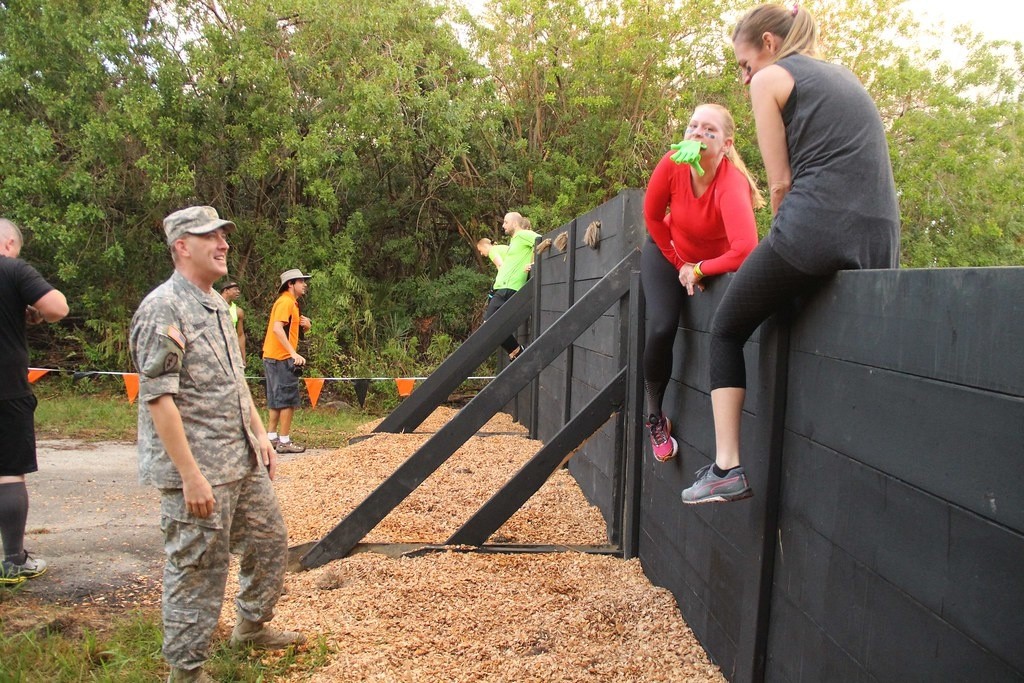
696,262,704,276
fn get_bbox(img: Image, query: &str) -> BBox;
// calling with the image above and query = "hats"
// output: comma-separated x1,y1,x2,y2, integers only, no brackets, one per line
221,280,239,291
276,269,312,295
162,205,237,245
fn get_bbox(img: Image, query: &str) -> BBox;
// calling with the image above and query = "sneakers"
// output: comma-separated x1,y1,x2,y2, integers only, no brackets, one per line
276,440,306,454
270,436,279,449
681,461,754,505
646,413,678,462
230,616,307,648
0,549,47,583
167,664,221,683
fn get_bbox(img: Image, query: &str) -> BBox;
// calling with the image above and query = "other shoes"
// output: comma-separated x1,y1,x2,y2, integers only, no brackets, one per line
510,345,525,363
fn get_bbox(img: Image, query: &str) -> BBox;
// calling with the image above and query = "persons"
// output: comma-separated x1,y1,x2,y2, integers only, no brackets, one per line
218,280,246,368
639,103,758,460
128,205,306,683
477,212,542,365
262,269,312,454
679,0,898,504
0,219,70,584
